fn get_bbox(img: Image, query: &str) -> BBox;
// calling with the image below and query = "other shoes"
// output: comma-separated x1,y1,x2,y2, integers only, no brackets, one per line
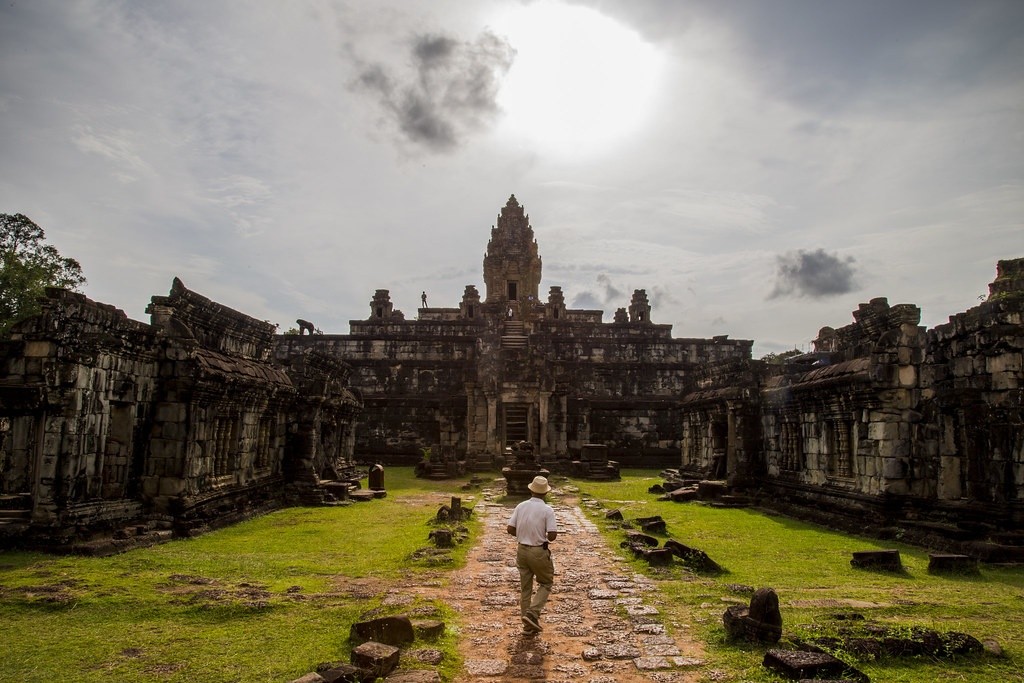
522,629,536,635
521,613,544,632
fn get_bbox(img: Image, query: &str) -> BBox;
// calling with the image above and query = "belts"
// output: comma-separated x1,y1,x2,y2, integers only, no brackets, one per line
518,543,544,548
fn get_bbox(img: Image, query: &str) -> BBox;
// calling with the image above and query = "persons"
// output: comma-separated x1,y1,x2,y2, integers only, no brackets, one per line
507,307,512,321
421,292,427,309
506,476,557,634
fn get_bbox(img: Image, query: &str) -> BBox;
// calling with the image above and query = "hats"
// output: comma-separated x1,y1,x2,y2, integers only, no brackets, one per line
528,475,552,494
